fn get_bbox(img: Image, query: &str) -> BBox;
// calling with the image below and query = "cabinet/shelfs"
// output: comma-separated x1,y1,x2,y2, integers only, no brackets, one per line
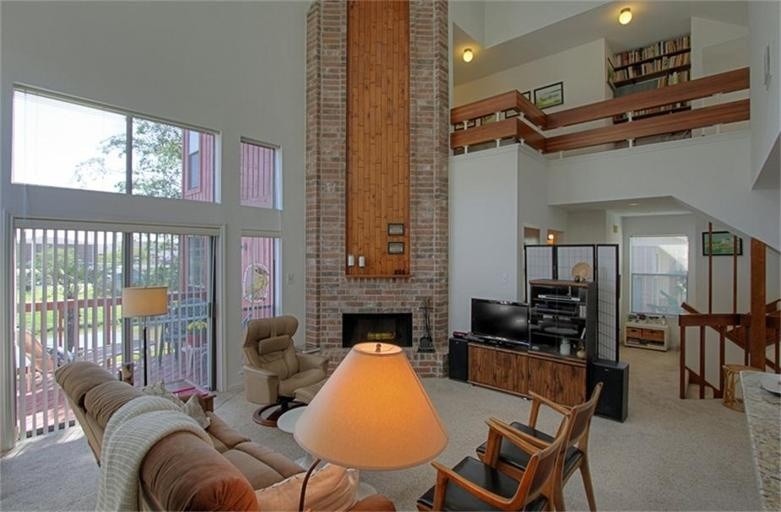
527,280,598,409
468,341,528,398
613,35,691,122
623,321,671,351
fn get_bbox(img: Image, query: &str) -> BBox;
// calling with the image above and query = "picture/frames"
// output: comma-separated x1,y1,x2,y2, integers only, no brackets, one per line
533,80,564,110
505,91,531,119
702,231,743,256
454,119,476,131
388,241,405,255
387,223,405,236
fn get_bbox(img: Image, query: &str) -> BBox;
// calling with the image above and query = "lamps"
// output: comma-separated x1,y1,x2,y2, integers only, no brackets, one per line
121,285,169,387
293,341,450,512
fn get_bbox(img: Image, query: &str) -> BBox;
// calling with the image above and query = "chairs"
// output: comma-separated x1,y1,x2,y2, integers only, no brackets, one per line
476,382,604,512
416,412,573,511
241,315,329,427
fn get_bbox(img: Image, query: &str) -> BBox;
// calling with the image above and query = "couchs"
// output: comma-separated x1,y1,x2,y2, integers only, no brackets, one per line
54,360,396,512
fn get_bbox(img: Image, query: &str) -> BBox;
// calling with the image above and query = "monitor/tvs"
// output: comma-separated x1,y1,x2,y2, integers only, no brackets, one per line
471,298,531,349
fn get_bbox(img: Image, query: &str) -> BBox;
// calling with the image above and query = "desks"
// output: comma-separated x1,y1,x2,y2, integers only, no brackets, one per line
739,371,781,511
164,377,217,413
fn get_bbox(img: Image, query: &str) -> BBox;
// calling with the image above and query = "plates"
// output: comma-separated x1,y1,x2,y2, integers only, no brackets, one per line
760,374,781,394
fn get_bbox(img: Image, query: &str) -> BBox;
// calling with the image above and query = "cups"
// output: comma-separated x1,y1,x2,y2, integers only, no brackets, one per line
560,339,571,356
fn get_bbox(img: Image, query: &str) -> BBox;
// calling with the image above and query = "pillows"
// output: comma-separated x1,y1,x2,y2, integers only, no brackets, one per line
253,462,359,512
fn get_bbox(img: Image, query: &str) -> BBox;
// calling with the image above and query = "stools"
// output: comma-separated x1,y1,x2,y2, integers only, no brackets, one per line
721,364,763,413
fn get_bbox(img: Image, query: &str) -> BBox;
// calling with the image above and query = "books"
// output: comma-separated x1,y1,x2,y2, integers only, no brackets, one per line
607,34,691,122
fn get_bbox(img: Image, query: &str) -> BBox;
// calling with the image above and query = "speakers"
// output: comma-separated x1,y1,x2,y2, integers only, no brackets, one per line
449,337,469,382
588,358,629,424
